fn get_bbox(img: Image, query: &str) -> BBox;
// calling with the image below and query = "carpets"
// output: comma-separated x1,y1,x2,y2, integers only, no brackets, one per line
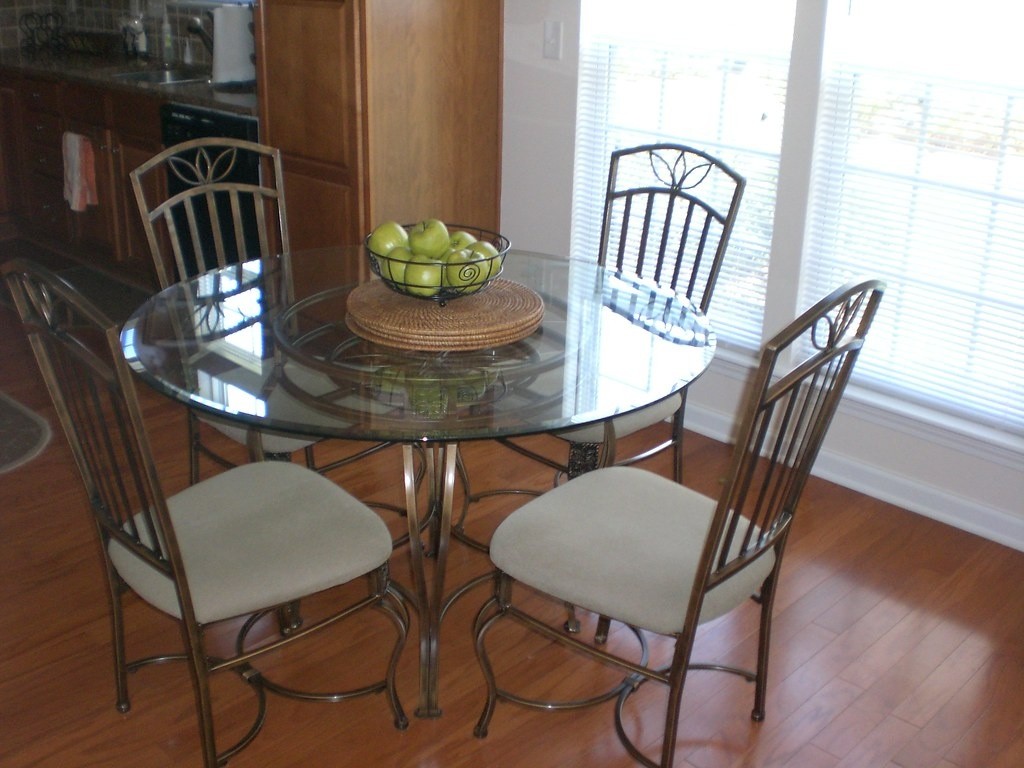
0,390,52,474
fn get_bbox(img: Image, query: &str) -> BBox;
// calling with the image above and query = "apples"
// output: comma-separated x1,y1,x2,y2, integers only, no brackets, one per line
404,256,446,296
447,248,488,293
440,248,457,286
381,247,412,288
409,219,450,258
368,220,410,258
450,231,478,251
466,241,501,277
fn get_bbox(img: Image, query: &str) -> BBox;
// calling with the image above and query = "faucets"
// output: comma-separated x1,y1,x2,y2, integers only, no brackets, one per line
186,9,214,57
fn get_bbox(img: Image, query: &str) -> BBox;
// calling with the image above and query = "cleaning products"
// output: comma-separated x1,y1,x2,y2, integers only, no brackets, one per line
160,3,174,62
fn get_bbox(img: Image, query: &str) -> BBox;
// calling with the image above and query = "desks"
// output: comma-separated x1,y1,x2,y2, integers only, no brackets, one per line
121,247,720,716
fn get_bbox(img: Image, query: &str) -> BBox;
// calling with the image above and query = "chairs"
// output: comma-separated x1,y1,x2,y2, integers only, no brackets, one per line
129,136,440,634
451,141,746,638
0,258,412,768
467,278,887,768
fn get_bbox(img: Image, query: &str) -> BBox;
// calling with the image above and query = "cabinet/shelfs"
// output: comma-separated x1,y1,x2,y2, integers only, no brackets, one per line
257,0,502,368
0,70,174,290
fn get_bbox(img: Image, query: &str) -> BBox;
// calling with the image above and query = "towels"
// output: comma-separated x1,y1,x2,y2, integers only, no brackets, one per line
60,131,99,212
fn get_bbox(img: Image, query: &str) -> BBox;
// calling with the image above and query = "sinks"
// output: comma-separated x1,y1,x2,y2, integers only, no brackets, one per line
110,67,208,85
